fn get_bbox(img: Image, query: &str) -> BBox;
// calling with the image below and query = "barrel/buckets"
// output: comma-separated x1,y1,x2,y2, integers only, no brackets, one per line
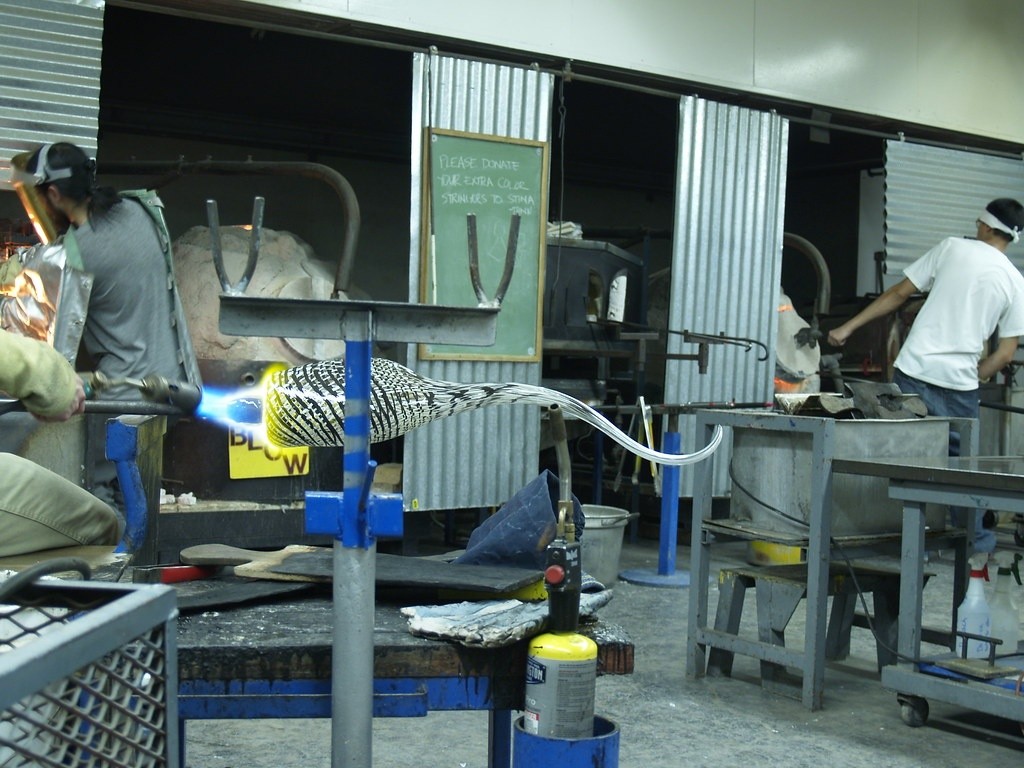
576,504,634,587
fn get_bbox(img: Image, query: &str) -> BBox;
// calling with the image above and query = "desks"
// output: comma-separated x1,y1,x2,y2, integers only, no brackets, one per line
829,453,1024,738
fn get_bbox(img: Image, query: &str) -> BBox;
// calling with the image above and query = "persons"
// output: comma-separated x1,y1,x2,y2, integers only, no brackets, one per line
825,198,1024,562
0,329,128,597
25,141,203,517
